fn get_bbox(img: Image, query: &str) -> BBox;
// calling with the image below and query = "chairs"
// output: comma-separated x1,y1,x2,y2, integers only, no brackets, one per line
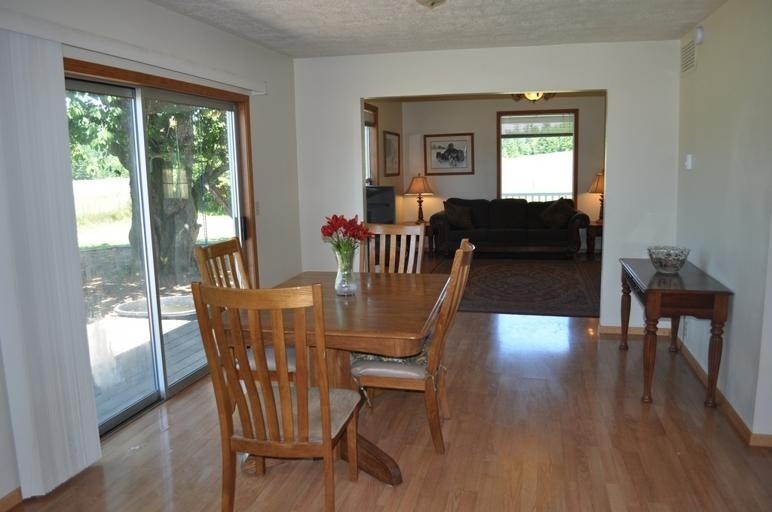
191,239,310,429
191,278,362,512
359,220,426,272
349,236,475,456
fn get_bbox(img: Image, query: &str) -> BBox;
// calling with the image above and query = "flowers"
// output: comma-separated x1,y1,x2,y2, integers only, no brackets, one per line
320,211,374,244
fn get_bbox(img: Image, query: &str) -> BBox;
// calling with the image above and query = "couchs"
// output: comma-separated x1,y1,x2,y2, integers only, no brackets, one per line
429,194,589,264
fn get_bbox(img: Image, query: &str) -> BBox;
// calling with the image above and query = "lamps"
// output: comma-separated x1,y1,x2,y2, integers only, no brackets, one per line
403,171,434,223
511,92,556,103
587,173,604,224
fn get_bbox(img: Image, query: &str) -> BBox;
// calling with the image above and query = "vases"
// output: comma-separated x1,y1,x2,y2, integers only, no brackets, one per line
328,245,356,294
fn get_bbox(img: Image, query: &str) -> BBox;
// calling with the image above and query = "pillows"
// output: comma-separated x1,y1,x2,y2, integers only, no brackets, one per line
440,199,474,230
539,196,577,229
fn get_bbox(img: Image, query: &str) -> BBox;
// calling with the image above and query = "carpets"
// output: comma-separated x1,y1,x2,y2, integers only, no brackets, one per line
433,250,602,316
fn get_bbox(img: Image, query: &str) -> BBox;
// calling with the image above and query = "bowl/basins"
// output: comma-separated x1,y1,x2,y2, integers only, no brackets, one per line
648,245,691,276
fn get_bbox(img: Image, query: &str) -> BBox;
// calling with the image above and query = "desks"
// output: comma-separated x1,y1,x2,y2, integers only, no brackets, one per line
585,221,603,261
618,255,735,411
222,272,454,487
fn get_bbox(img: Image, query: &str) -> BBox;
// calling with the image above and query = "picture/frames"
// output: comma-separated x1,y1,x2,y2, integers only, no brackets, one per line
423,131,474,176
383,129,402,177
